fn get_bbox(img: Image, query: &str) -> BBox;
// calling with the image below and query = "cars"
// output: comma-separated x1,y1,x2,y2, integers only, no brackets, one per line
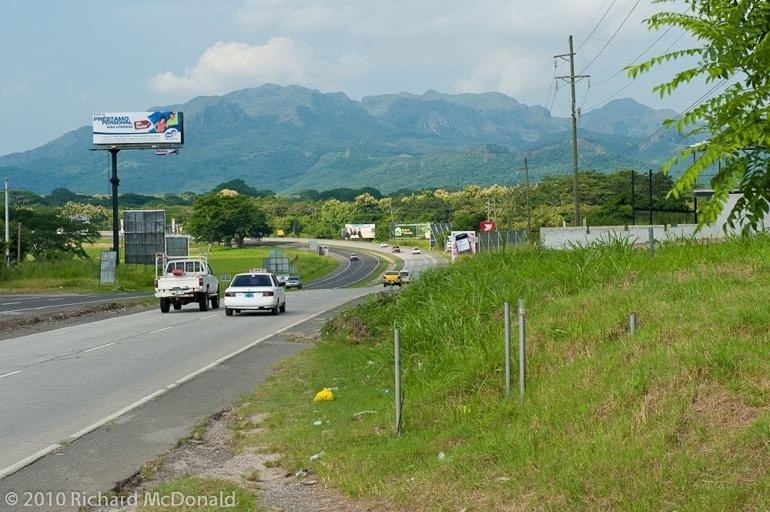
412,246,421,254
392,245,400,253
285,276,302,289
223,272,286,316
455,233,472,255
349,253,358,261
379,242,388,247
383,270,410,287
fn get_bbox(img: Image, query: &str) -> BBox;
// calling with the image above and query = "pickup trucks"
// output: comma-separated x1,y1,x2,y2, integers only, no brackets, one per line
154,260,220,312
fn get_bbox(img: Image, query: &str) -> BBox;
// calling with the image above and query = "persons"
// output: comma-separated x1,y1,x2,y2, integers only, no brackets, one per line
167,112,178,126
155,117,168,133
